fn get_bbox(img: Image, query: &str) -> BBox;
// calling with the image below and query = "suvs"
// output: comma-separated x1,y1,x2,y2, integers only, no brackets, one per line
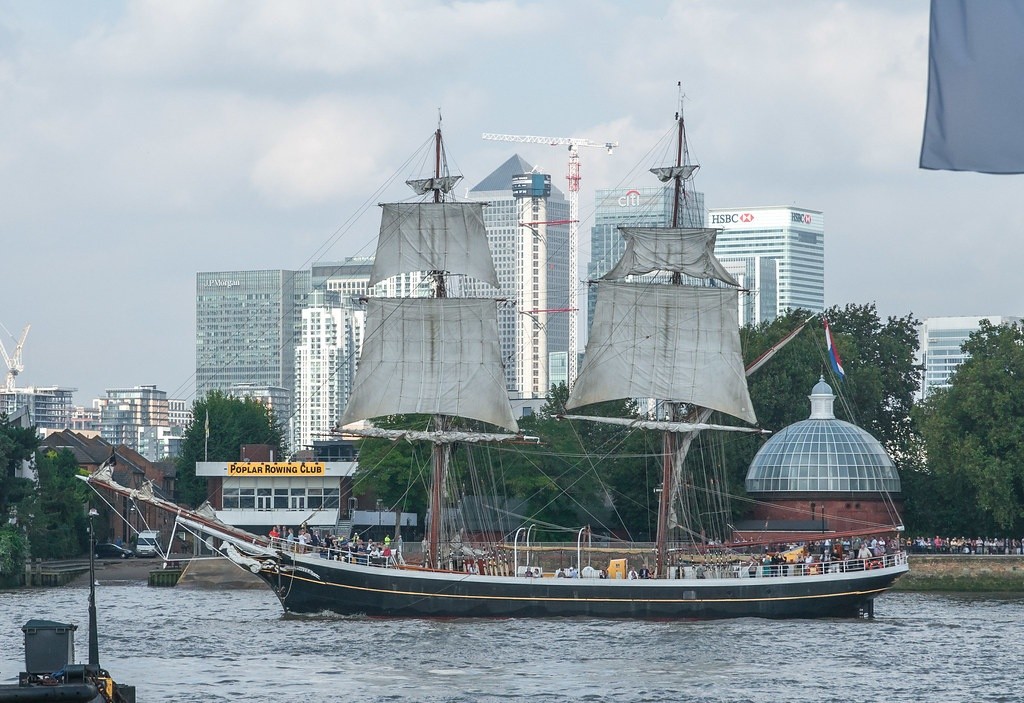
93,543,134,560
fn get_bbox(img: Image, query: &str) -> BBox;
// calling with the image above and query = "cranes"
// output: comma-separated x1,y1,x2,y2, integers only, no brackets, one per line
480,131,616,397
0,321,31,389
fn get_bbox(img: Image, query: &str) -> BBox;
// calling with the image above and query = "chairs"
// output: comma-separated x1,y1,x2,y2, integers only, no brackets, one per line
380,549,397,568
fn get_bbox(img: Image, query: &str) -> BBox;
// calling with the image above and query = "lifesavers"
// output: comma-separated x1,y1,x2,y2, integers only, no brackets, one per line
807,564,820,575
869,560,882,571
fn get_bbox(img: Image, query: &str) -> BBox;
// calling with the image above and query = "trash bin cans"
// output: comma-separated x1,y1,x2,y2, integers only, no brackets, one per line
22,619,78,675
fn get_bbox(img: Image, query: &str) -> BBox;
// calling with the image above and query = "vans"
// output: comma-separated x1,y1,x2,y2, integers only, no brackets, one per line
135,529,161,558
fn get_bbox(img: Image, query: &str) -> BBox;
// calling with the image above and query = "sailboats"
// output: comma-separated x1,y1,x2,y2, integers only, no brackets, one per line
73,77,910,621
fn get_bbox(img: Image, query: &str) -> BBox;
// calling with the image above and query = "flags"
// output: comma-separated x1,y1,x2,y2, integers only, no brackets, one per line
824,318,845,382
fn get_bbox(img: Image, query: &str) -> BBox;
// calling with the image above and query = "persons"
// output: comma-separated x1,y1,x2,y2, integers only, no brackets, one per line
674,565,685,579
115,536,122,548
753,536,906,575
269,522,403,565
106,537,112,544
696,565,705,579
525,565,655,580
907,535,1023,555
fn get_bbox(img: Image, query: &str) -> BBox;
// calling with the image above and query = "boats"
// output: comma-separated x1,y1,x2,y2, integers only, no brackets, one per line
0,509,137,703
